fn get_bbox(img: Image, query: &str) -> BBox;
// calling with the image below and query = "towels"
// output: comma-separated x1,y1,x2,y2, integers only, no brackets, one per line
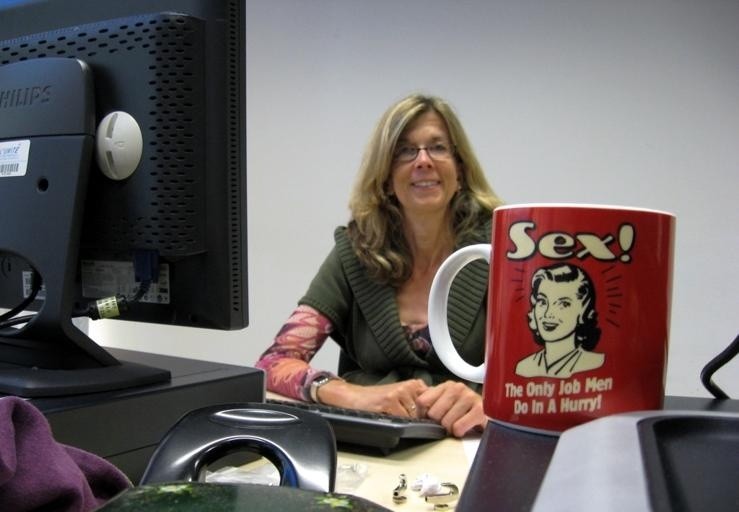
1,395,131,510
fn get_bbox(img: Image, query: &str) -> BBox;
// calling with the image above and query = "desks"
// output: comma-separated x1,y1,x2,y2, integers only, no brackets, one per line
205,395,490,511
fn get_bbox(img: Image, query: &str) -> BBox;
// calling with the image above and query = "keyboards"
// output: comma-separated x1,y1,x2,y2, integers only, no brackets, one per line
265,398,448,454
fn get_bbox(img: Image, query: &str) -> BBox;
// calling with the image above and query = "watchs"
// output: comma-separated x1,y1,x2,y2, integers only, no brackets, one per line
310,374,338,404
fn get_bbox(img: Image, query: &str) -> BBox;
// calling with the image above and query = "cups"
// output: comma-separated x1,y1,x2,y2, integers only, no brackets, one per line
425,200,680,438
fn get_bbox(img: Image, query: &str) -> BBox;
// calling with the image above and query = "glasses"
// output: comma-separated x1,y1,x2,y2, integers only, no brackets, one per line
392,140,459,163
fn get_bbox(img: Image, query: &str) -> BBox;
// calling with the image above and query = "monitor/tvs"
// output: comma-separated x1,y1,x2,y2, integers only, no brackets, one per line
0,0,250,397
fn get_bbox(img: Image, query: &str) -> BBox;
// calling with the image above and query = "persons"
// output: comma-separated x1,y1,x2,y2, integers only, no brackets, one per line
255,93,514,442
512,260,606,379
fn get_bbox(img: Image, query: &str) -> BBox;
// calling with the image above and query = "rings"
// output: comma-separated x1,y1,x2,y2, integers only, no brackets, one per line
407,405,417,413
